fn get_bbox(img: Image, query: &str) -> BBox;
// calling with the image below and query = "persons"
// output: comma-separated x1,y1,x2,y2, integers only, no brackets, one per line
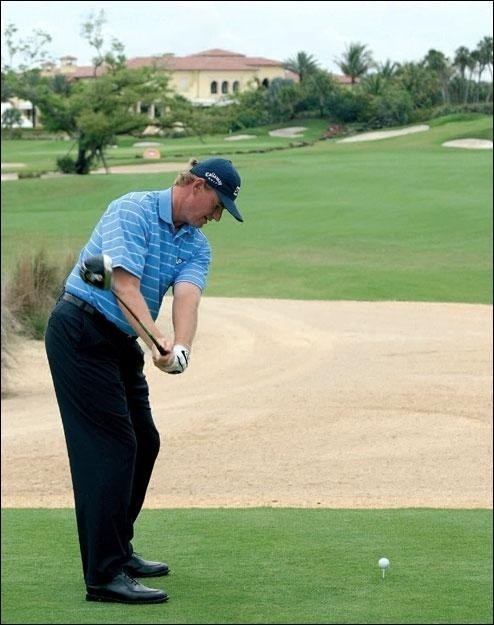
45,158,242,604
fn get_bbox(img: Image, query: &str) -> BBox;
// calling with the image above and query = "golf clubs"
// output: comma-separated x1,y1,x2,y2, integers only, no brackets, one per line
79,256,168,357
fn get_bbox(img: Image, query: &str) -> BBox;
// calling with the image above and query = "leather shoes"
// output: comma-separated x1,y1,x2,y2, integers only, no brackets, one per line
86,570,168,604
123,551,170,578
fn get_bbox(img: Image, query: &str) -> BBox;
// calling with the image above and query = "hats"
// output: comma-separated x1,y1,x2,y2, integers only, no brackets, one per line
190,158,244,224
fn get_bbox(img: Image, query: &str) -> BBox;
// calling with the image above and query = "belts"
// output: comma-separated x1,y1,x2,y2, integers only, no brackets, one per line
62,293,95,316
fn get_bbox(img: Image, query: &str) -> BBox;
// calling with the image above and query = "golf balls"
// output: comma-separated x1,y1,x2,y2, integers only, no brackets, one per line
379,558,389,568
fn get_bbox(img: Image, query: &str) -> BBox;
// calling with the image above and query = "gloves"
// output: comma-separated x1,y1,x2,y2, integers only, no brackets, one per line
168,344,191,374
